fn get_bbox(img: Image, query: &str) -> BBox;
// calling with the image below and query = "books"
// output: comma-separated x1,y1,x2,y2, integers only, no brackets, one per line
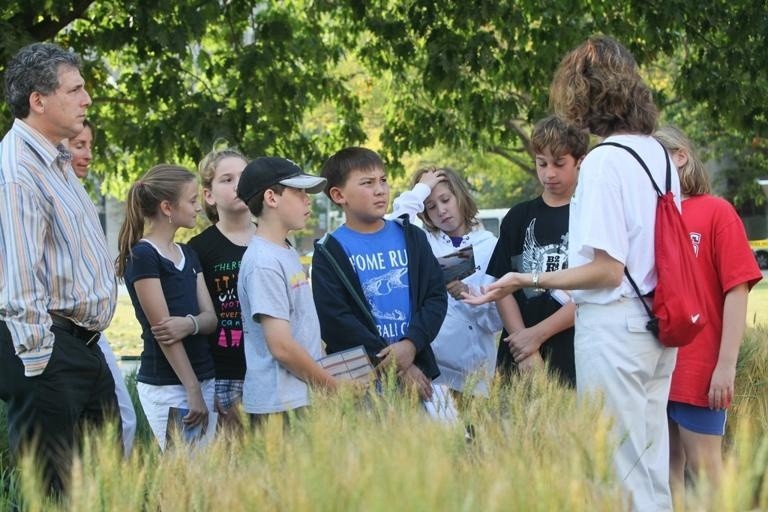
324,355,371,379
315,345,366,370
351,372,378,387
162,404,220,456
435,242,476,286
335,364,373,382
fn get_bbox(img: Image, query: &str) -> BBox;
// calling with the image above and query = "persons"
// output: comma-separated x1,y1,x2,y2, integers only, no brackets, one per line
1,39,127,511
64,116,139,470
187,138,271,442
644,121,762,512
459,34,686,512
481,114,591,401
389,165,508,445
232,154,355,445
111,163,220,458
307,142,448,419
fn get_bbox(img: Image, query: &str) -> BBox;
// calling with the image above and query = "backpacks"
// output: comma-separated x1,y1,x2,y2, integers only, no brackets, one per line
590,137,710,348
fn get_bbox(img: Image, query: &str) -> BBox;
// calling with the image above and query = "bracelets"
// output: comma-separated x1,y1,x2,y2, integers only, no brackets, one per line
530,271,547,295
185,314,199,338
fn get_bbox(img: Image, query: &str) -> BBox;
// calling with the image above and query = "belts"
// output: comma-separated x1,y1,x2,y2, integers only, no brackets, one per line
47,310,102,348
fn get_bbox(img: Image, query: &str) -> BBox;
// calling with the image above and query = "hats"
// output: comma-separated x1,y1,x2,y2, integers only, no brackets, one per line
237,156,329,205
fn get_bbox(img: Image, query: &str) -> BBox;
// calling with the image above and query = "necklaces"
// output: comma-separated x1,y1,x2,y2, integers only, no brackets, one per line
217,221,252,247
